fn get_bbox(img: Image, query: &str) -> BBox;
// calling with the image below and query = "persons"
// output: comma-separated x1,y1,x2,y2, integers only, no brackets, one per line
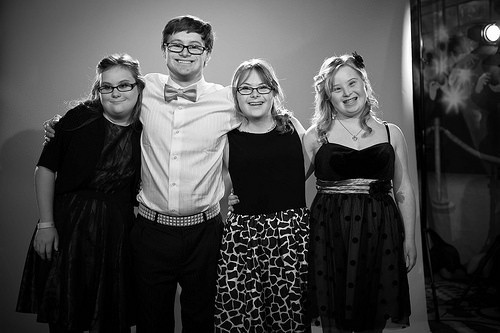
227,51,419,333
42,15,247,333
213,60,313,333
14,55,145,333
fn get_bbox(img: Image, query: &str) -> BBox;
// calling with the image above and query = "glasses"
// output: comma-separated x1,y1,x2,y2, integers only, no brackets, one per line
97,82,138,94
238,84,274,95
164,41,207,55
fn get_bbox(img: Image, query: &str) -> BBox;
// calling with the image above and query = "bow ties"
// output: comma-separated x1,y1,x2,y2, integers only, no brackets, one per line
163,83,198,102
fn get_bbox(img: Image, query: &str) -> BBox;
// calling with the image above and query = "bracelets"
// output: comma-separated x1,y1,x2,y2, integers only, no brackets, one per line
37,221,54,229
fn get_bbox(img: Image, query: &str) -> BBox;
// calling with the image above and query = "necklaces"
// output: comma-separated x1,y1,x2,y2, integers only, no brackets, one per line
266,122,276,132
336,117,371,140
